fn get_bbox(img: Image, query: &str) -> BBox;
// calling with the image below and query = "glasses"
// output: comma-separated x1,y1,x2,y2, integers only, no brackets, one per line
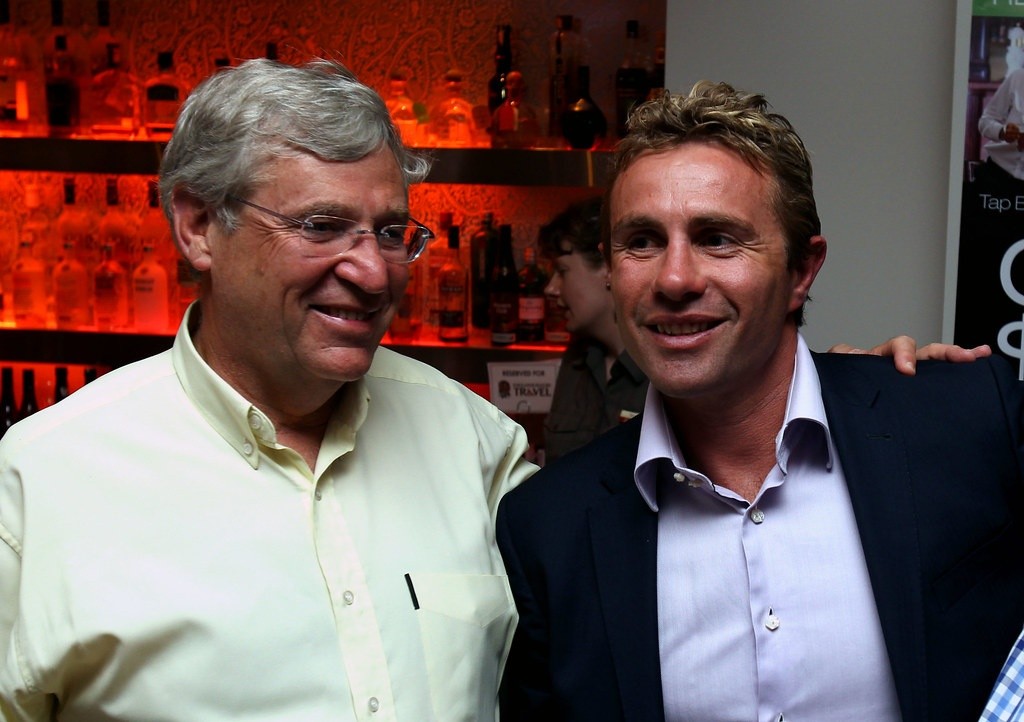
226,194,434,265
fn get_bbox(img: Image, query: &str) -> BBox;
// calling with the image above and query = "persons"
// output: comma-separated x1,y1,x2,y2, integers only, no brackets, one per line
536,197,651,464
0,54,991,722
496,76,1024,722
978,68,1024,181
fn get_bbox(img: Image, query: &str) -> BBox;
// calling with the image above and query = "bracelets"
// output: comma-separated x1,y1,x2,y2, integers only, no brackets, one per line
1004,125,1006,133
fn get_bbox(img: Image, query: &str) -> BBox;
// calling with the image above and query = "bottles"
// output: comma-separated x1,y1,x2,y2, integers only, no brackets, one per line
1,0,669,464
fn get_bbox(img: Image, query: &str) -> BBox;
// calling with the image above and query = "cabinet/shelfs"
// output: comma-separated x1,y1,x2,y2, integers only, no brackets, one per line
0,114,627,384
